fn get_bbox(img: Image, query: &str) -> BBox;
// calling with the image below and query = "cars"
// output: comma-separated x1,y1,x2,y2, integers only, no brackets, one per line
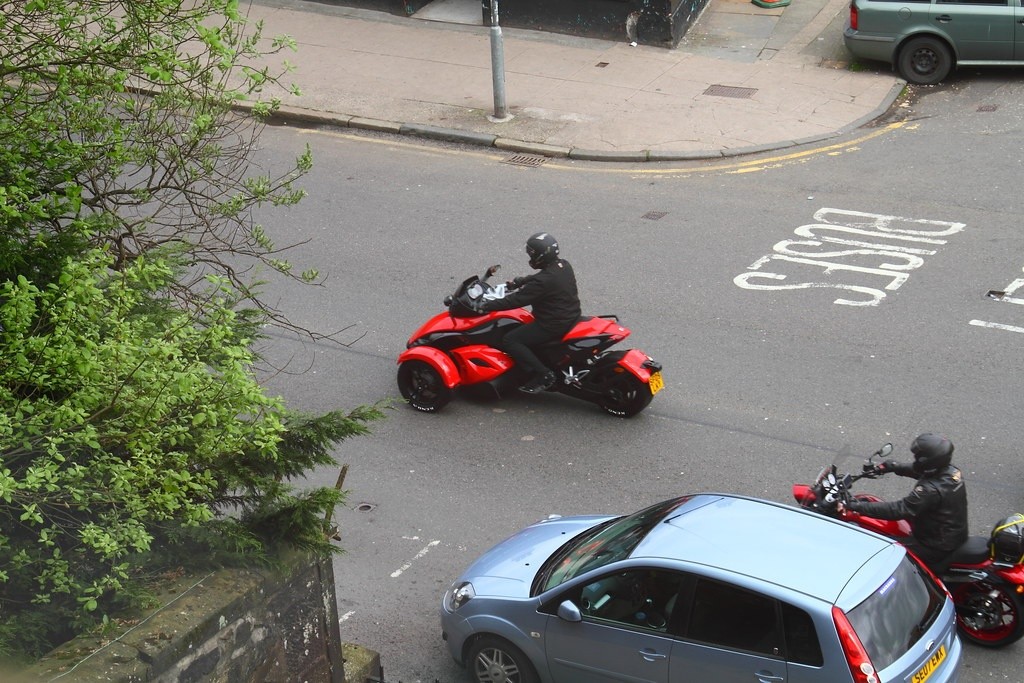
840,0,1024,90
437,493,968,683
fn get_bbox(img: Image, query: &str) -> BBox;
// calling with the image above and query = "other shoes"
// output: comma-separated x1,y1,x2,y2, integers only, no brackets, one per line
518,367,558,394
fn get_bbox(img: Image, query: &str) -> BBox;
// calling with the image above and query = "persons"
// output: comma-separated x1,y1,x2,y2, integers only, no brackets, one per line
840,433,969,573
477,232,581,394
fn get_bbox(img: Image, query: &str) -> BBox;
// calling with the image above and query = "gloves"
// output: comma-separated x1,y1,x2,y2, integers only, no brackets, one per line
474,298,489,313
843,501,864,516
513,276,526,287
881,459,900,474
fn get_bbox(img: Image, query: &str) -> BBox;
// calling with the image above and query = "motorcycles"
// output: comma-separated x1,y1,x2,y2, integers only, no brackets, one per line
794,444,1024,650
397,264,666,419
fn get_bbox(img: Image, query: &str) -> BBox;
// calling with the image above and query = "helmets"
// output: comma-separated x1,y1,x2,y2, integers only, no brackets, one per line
910,433,955,477
525,231,559,269
